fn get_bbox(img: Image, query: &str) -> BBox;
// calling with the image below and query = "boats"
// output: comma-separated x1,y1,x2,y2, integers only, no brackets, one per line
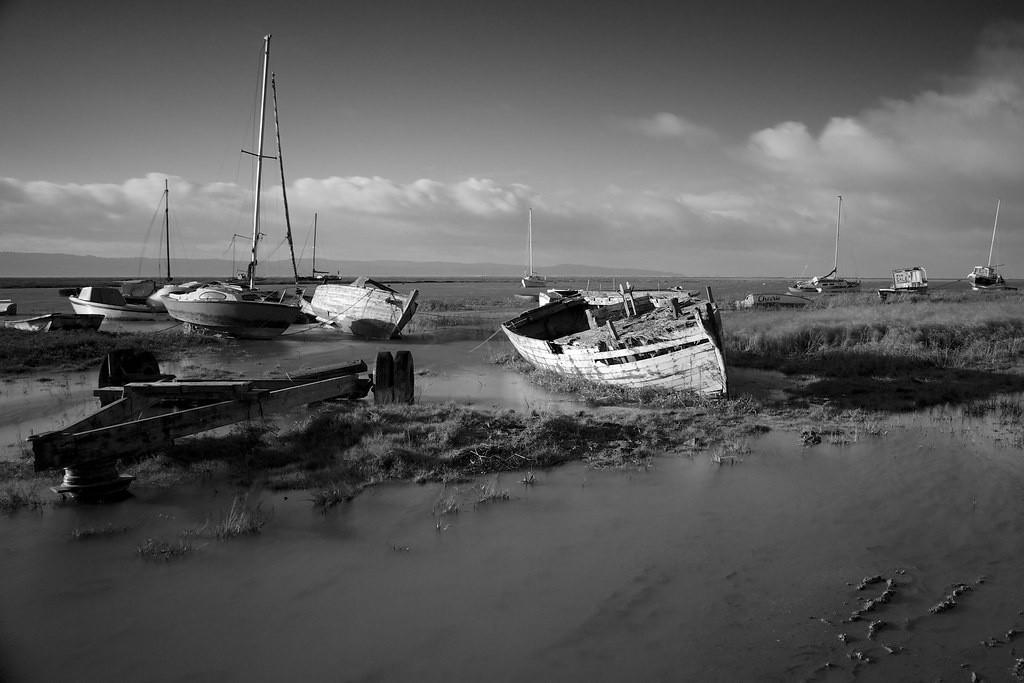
539,282,701,309
877,267,928,304
311,276,419,341
502,281,728,396
745,293,814,306
5,313,105,332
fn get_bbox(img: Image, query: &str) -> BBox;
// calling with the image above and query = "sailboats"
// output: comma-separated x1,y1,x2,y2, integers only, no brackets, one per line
160,33,300,338
968,198,1006,290
521,209,547,288
69,178,230,321
788,195,862,293
